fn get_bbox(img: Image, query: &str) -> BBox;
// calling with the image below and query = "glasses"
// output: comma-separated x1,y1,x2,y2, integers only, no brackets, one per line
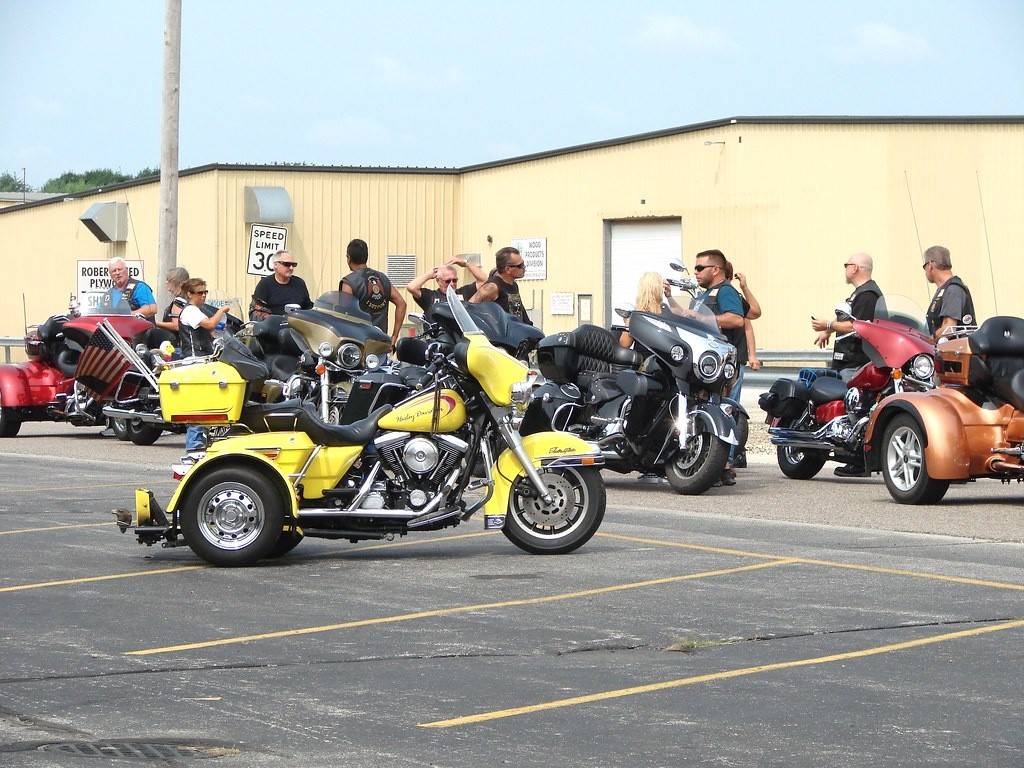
504,262,524,269
844,263,856,268
273,260,298,268
189,289,208,297
438,276,458,283
922,260,936,270
694,264,722,272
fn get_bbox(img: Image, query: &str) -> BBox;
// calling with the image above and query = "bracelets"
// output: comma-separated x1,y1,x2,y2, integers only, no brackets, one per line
462,259,468,266
827,320,832,331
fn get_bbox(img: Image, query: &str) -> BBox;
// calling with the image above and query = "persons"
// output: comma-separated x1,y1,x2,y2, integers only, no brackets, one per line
249,249,315,322
811,251,890,478
619,270,685,483
178,277,230,453
921,246,979,484
680,249,761,487
95,257,157,329
155,267,188,349
339,239,407,350
406,257,488,332
468,247,535,326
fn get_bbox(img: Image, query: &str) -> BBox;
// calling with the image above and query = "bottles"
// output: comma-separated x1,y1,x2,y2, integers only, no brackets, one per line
175,347,182,355
259,383,281,403
213,312,227,339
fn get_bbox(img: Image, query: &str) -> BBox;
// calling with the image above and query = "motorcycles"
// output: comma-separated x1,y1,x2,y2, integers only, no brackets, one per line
1,291,145,442
111,283,608,564
104,283,751,481
758,291,1024,504
519,259,740,497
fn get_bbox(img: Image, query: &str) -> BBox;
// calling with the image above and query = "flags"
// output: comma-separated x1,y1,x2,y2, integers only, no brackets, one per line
72,318,131,403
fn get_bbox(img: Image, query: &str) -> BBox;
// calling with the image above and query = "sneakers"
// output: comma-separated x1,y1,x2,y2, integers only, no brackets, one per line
100,428,116,437
834,463,871,477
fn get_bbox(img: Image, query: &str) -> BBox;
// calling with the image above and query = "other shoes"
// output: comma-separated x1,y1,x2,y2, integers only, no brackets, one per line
637,471,658,483
721,468,737,485
187,449,206,459
161,429,172,436
659,474,668,483
726,457,747,467
